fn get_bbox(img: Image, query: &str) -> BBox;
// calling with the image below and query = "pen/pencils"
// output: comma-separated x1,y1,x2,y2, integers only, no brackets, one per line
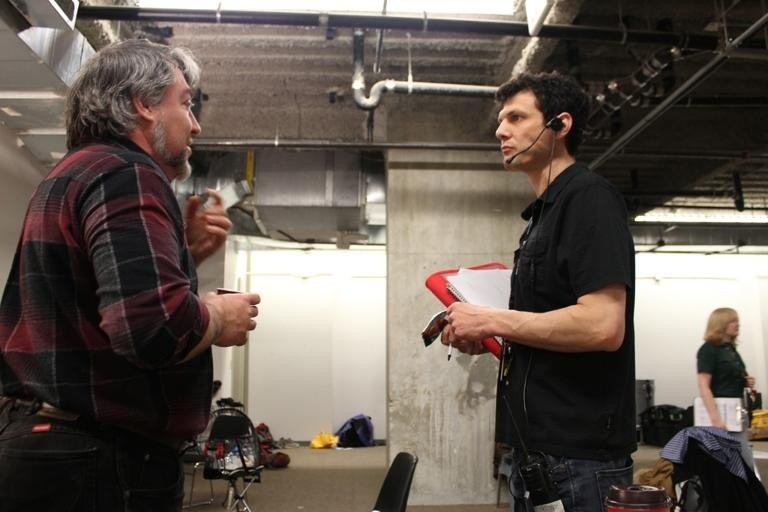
447,344,452,360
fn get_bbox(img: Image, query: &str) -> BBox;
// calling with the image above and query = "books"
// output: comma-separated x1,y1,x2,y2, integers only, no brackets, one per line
424,262,513,360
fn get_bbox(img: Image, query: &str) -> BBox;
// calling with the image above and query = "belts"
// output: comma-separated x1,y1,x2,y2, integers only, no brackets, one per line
1,392,197,455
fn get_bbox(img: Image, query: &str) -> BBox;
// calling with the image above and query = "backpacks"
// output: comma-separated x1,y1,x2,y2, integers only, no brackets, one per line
335,414,374,447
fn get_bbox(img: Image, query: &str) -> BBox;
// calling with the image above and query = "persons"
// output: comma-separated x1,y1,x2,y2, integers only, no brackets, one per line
440,71,639,512
693,306,756,432
0,38,262,512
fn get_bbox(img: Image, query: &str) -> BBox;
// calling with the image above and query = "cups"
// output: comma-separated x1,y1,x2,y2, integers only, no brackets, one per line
604,483,671,512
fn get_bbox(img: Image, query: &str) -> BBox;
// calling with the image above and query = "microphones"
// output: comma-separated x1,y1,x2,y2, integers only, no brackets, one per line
506,116,557,164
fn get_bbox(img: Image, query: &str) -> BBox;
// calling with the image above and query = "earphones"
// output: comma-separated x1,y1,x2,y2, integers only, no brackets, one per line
551,118,563,131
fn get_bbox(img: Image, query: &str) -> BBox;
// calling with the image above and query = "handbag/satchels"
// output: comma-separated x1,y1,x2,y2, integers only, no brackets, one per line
671,437,768,512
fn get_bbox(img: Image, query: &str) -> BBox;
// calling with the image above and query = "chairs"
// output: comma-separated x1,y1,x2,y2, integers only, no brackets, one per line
372,451,418,512
204,408,265,512
183,411,214,509
